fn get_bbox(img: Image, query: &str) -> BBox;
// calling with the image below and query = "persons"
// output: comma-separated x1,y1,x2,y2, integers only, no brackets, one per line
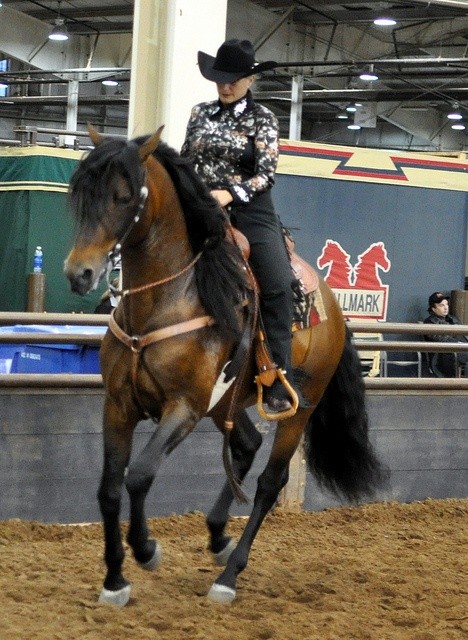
180,39,314,411
425,292,468,380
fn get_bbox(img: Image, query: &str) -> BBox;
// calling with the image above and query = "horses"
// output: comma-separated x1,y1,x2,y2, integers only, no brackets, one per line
61,120,389,613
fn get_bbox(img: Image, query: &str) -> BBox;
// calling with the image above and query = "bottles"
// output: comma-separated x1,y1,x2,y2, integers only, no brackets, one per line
34,247,42,272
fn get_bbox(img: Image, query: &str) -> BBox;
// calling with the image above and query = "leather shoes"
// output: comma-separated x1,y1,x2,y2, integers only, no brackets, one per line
266,395,291,412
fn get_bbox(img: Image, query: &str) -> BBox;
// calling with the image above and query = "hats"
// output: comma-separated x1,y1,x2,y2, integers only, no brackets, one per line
428,292,449,304
197,39,277,83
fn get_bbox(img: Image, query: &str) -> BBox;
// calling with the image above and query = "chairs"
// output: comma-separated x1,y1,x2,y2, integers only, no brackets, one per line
347,317,387,377
417,319,468,377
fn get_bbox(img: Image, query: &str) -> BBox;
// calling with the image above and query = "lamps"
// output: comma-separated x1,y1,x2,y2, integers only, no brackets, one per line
101,77,120,87
359,66,379,83
447,101,462,120
347,124,361,131
48,16,71,40
450,124,466,131
335,110,348,119
346,101,357,112
373,8,397,28
